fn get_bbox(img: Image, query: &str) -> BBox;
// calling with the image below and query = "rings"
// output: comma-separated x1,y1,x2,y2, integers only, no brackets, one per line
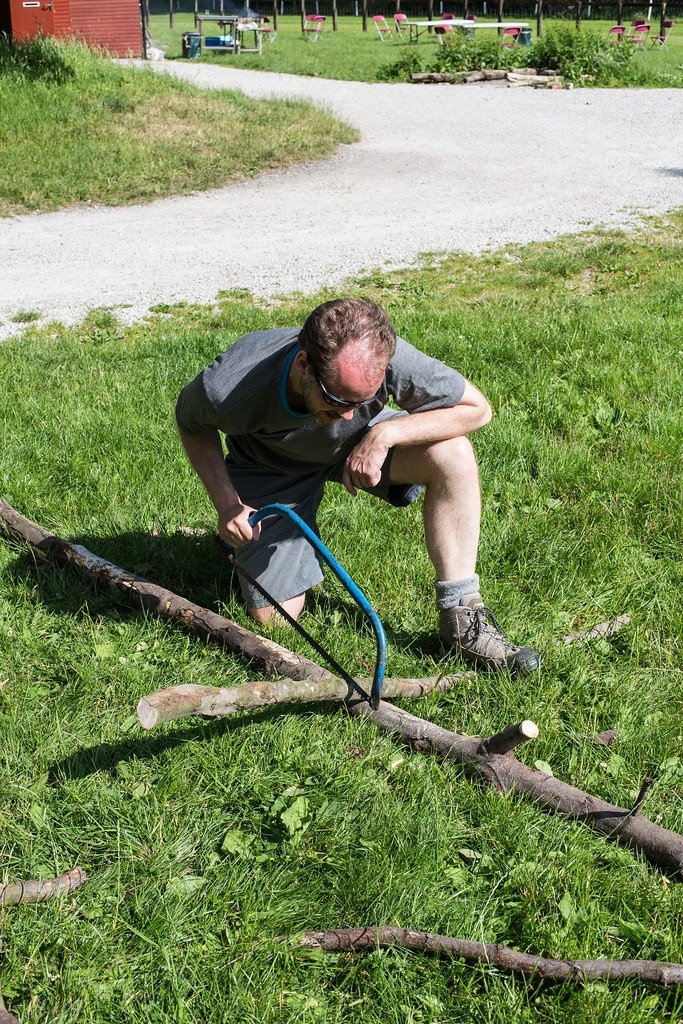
230,535,235,539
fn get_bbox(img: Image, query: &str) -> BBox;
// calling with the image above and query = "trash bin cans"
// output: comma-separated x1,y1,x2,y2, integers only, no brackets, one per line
181,31,203,59
514,27,533,46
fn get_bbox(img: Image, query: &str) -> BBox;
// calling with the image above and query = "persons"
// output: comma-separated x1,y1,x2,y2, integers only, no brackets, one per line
173,297,544,675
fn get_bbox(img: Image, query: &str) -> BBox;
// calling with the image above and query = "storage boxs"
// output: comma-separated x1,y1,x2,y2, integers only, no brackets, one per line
205,36,233,48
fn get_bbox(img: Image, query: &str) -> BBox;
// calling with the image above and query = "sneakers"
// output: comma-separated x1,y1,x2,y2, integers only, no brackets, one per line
439,593,541,679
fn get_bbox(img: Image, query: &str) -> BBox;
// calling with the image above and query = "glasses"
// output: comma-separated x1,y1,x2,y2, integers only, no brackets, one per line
307,359,381,409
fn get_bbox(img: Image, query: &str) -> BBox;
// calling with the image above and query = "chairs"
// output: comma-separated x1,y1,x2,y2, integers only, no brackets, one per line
371,14,393,41
607,19,675,51
302,15,326,43
434,15,475,45
394,14,412,38
500,27,522,49
255,15,278,42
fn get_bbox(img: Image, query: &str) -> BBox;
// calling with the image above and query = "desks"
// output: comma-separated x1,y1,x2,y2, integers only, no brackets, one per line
403,19,473,43
234,27,266,55
195,12,237,53
462,22,529,48
